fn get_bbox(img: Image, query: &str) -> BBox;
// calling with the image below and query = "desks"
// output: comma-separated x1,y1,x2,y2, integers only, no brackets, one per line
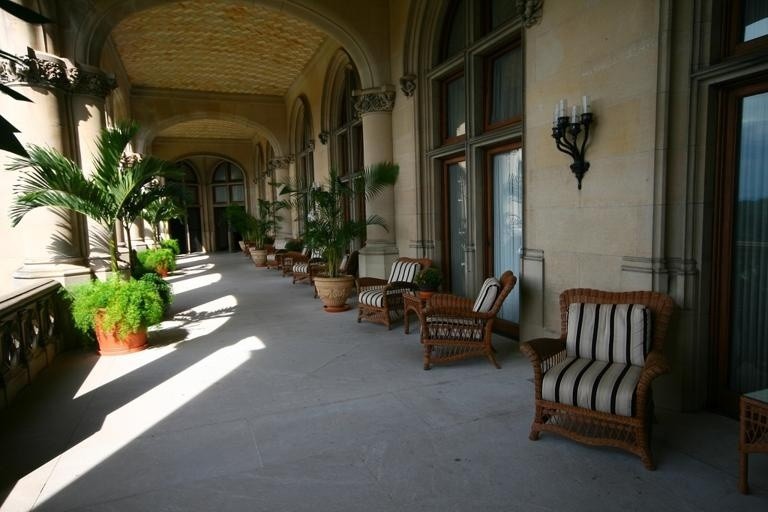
402,291,449,344
737,389,768,495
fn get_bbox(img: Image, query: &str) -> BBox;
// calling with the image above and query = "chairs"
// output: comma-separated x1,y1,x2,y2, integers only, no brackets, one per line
420,270,517,370
354,257,433,331
519,288,677,470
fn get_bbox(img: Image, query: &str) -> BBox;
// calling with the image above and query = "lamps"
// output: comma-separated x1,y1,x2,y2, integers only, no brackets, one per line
550,95,597,190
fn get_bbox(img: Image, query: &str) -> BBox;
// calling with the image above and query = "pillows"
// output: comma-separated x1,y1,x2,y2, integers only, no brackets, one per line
472,277,502,312
387,261,421,284
566,302,651,368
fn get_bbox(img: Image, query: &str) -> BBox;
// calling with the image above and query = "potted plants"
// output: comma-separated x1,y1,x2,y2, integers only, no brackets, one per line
414,264,443,299
4,114,188,356
226,161,400,312
